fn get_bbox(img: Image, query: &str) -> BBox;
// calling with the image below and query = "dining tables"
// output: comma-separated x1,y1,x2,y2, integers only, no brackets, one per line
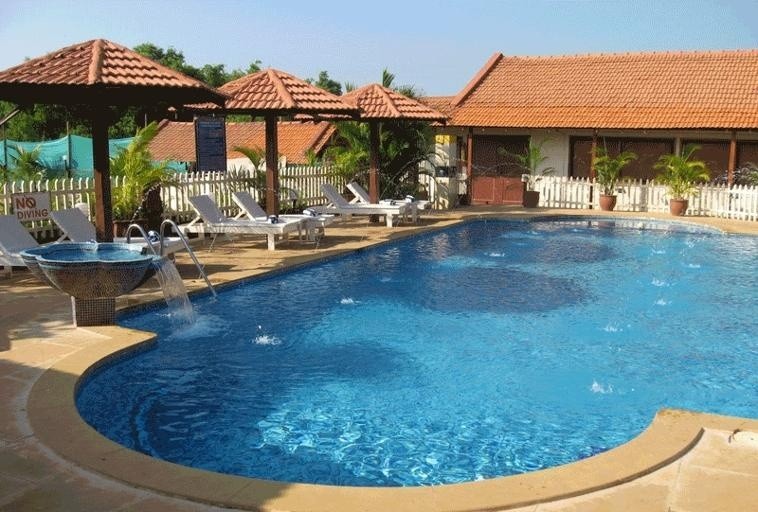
508,142,708,215
111,132,154,236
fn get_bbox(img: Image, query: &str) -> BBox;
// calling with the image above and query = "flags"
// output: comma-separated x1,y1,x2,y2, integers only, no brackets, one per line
598,219,617,234
